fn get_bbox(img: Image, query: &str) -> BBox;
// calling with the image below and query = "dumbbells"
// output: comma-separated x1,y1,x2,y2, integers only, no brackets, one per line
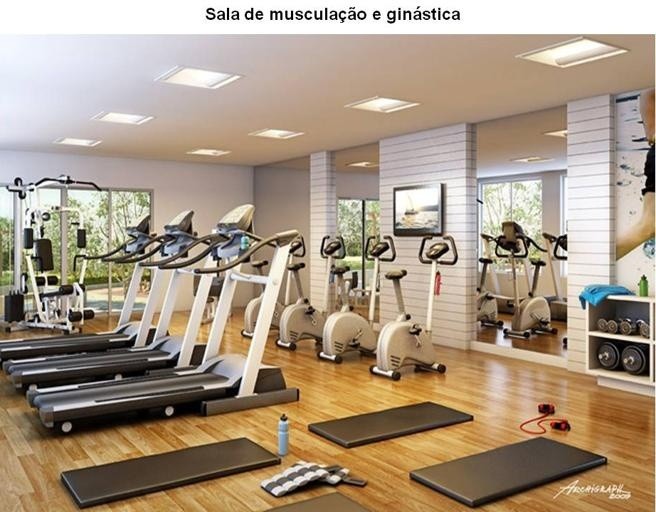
596,316,650,375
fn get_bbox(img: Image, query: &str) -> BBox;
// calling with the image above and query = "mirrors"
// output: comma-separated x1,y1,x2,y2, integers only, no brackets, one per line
471,105,568,371
330,141,379,332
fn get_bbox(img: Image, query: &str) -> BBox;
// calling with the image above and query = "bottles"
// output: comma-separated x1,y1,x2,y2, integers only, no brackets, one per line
638,274,648,297
278,412,289,455
434,270,441,295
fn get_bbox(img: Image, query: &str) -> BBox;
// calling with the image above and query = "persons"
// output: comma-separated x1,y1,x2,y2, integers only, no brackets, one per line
610,87,654,266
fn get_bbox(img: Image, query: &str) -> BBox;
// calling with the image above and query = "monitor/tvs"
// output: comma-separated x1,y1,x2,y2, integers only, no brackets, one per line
393,182,442,237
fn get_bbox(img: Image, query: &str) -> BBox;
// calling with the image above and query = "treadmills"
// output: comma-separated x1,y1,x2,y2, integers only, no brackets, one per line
1,204,299,433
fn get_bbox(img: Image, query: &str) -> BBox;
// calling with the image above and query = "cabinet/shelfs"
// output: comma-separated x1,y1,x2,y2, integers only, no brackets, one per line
585,295,656,397
